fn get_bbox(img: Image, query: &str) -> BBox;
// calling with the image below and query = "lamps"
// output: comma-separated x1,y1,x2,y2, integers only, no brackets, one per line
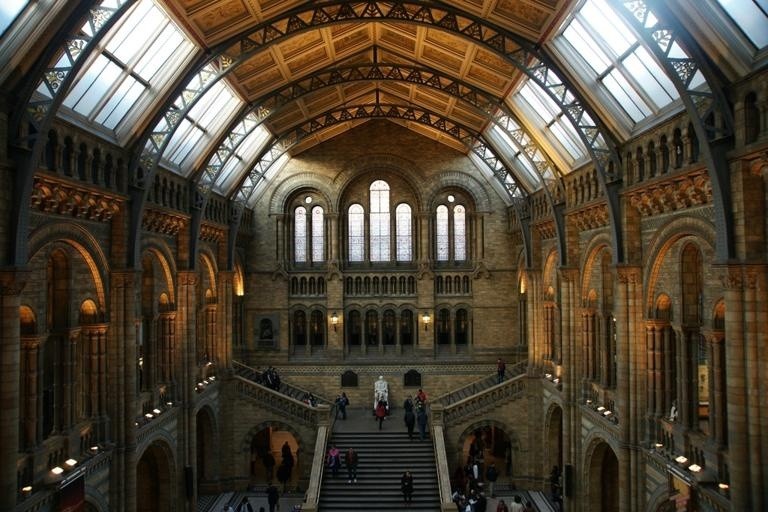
421,310,431,332
329,311,339,332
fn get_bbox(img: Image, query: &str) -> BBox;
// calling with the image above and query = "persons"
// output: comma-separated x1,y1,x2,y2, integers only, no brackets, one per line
669,400,678,422
200,352,208,364
